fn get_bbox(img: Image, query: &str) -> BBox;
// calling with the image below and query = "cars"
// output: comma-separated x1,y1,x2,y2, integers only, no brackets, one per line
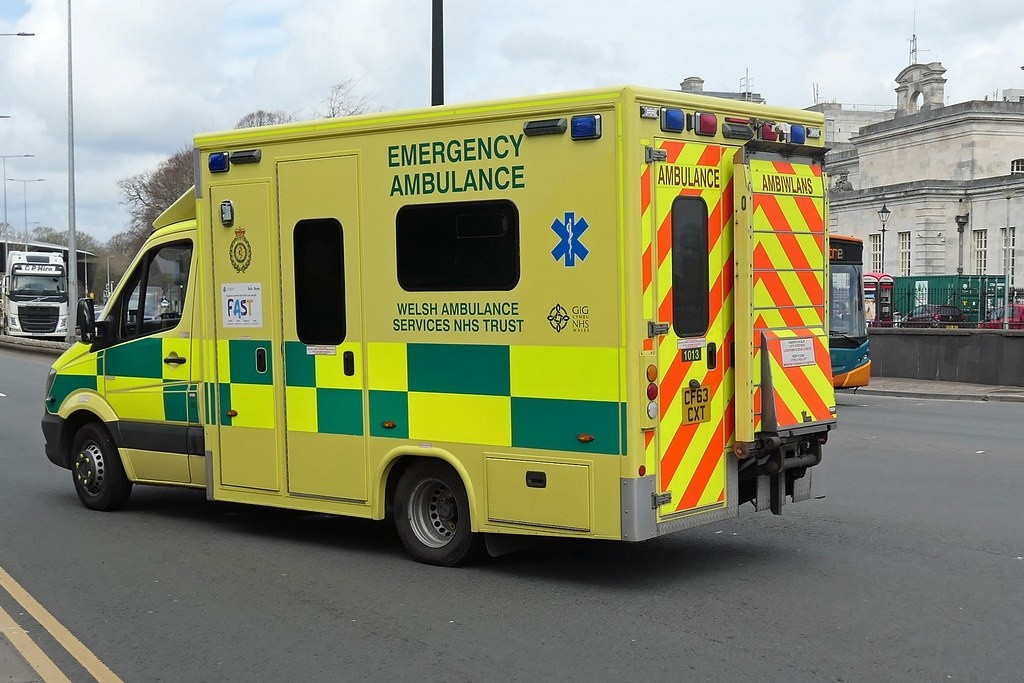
978,304,1024,329
899,304,968,329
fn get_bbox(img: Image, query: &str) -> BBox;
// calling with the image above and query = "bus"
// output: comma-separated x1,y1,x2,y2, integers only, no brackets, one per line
830,233,870,390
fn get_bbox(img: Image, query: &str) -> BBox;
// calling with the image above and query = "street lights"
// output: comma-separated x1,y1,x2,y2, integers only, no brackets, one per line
7,178,46,251
107,254,114,292
879,202,891,274
2,153,35,274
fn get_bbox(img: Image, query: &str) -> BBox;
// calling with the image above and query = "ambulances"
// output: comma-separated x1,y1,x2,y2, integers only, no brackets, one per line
37,83,839,570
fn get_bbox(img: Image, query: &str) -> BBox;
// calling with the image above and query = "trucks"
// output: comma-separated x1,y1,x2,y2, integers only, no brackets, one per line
0,250,70,342
